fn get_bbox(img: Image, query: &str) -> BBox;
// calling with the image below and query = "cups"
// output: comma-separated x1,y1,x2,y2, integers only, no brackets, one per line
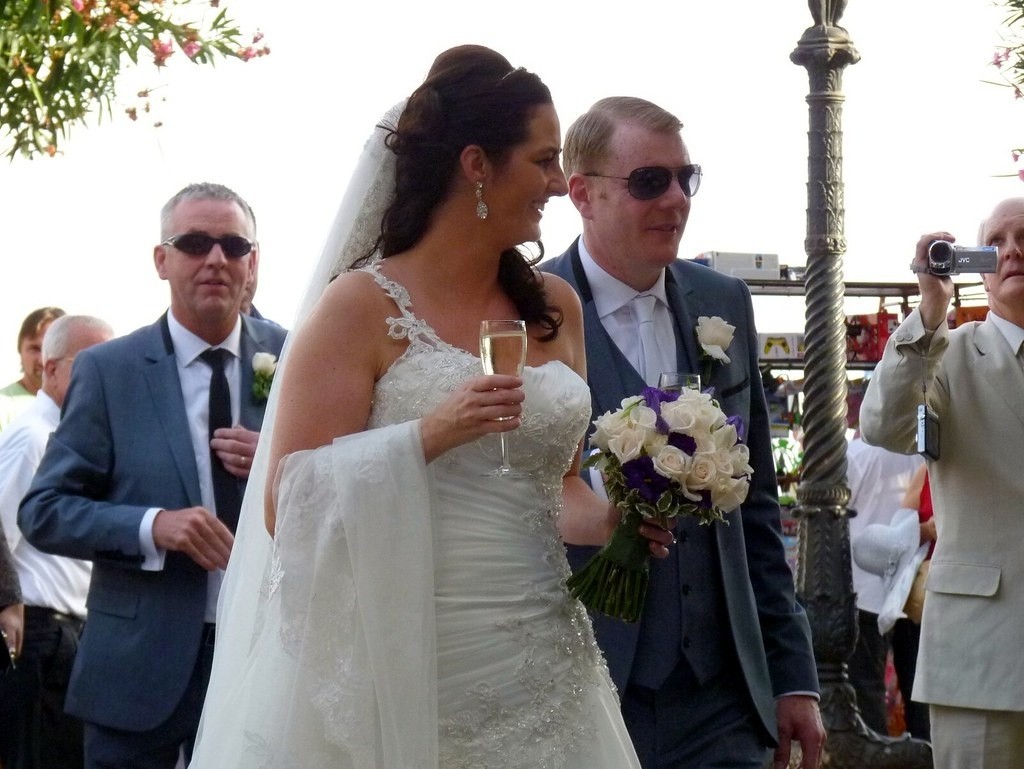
656,372,702,394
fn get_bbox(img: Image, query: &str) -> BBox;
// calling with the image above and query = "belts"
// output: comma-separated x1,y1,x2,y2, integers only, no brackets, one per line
23,604,85,634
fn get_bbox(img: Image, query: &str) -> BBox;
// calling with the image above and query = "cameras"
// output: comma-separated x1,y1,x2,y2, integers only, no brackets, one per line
915,404,941,462
928,240,1000,276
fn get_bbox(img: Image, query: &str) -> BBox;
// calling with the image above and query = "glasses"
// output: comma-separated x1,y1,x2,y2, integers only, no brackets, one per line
581,165,702,199
162,233,253,257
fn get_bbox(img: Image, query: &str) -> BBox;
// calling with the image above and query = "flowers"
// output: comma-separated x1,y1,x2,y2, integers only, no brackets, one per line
253,352,277,405
695,316,736,381
565,379,754,624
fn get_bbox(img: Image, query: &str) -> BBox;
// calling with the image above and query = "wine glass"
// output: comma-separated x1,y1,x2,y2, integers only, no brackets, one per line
479,319,531,480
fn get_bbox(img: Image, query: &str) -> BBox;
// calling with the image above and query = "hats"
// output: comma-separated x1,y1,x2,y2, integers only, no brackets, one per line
853,507,920,594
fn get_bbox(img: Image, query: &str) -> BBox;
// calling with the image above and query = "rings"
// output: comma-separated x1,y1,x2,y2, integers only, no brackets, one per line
671,539,677,544
241,456,246,466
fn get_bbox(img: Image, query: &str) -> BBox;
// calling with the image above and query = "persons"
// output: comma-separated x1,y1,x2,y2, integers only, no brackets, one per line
192,46,676,767
17,183,290,768
538,97,826,769
0,306,114,769
848,195,1024,769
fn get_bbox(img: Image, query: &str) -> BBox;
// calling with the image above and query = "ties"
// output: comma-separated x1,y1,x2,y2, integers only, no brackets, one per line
199,349,243,537
630,296,665,388
